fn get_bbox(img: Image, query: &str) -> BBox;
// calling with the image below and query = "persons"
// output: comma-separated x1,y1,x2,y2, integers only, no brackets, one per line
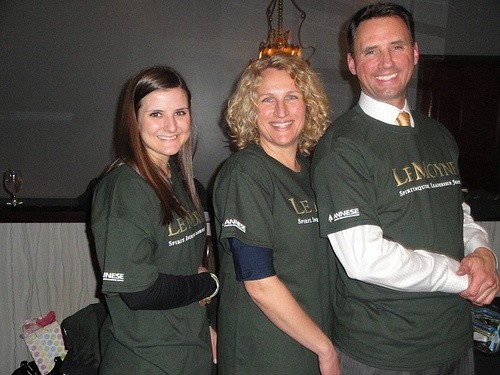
307,1,500,375
88,65,218,375
211,52,346,375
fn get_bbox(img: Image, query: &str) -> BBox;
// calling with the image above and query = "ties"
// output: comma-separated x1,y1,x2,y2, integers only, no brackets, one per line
396,112,410,127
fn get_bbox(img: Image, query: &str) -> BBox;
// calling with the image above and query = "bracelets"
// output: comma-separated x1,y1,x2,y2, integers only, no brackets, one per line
199,272,220,306
